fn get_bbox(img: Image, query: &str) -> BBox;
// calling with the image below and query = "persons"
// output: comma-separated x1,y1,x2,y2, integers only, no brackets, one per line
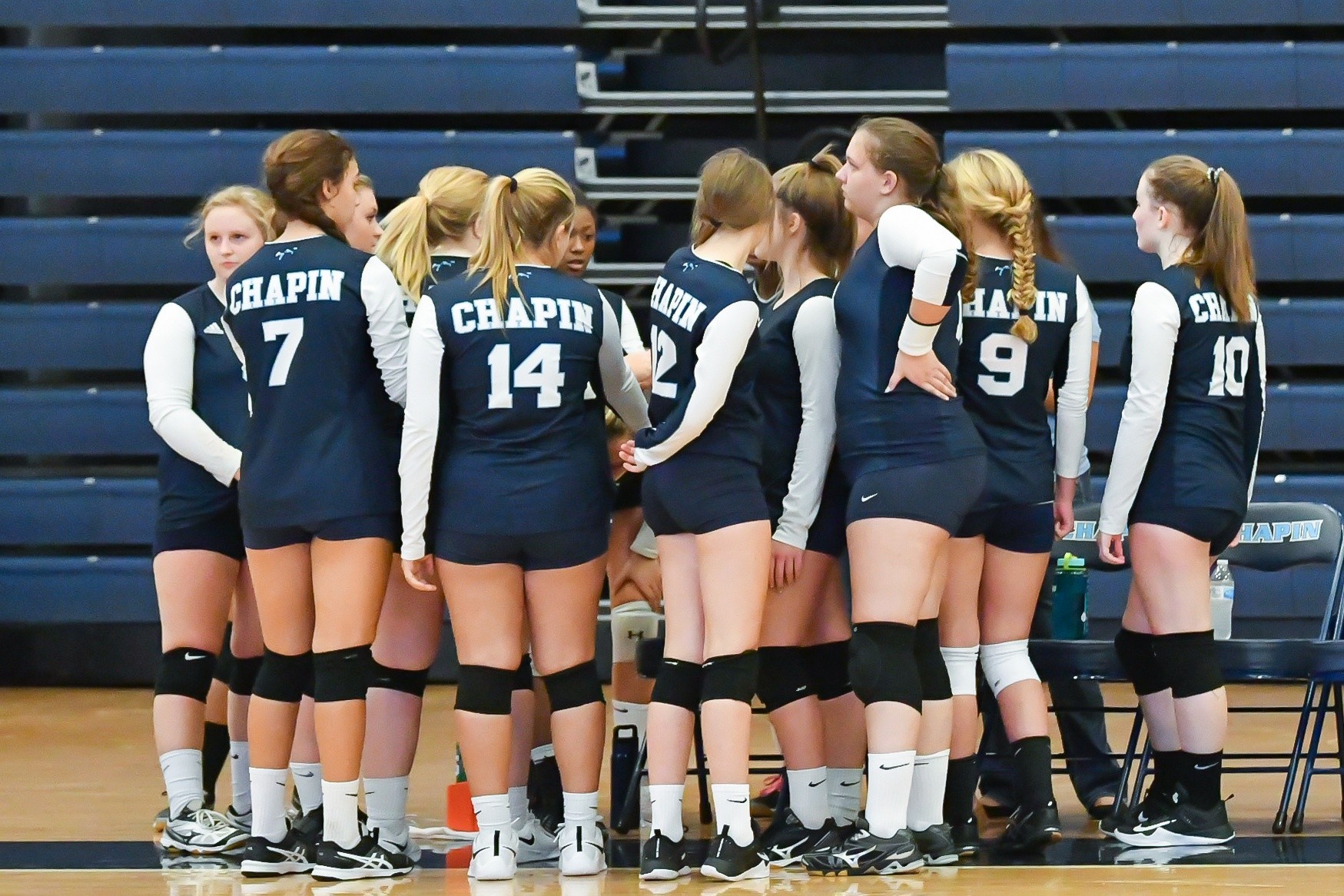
621,149,772,883
801,120,991,876
223,129,418,881
138,116,1124,882
1091,152,1267,848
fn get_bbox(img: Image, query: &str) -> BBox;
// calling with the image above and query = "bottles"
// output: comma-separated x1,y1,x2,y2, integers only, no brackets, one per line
456,741,467,783
1210,559,1235,641
1052,552,1089,639
612,724,640,830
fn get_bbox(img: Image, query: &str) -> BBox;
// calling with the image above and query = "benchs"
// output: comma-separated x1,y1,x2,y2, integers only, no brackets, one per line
946,1,1344,624
0,0,600,628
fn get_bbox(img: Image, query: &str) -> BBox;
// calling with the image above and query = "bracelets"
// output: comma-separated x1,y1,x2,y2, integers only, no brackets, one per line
896,310,942,358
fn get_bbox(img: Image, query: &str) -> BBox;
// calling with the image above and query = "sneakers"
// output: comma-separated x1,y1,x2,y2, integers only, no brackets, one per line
800,816,925,875
516,809,563,862
159,797,250,854
699,824,771,881
312,827,415,880
293,802,367,839
553,814,608,876
759,807,860,867
467,816,521,880
1098,786,1236,847
361,823,422,862
1087,803,1129,821
154,789,215,831
994,799,1065,856
907,821,959,865
639,825,693,880
224,804,297,834
241,823,318,876
952,816,981,856
977,802,1020,819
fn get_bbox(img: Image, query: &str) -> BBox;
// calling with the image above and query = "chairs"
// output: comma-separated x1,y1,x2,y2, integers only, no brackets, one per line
1027,500,1343,835
